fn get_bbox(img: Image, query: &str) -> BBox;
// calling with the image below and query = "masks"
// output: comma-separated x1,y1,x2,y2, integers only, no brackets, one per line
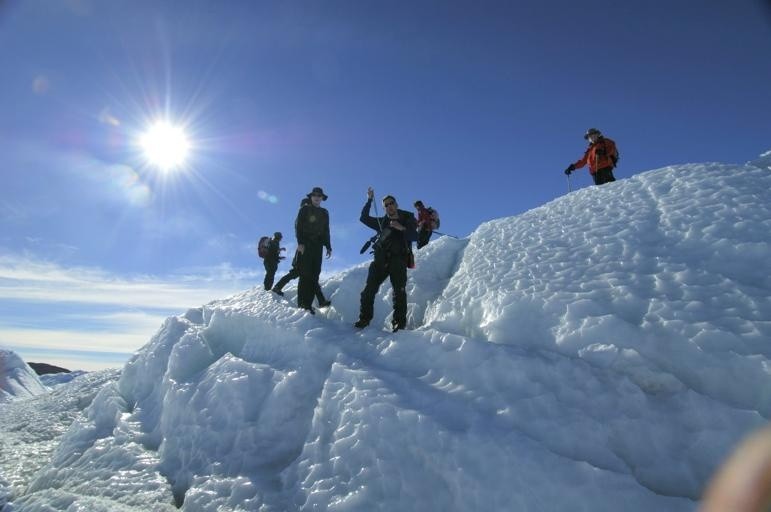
588,134,598,142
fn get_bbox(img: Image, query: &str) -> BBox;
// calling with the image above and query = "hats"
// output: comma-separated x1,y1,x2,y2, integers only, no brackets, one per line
414,200,423,207
584,127,600,137
274,231,282,239
306,186,328,202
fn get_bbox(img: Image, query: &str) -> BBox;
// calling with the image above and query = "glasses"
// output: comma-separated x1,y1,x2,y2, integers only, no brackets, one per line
382,202,395,208
311,192,322,198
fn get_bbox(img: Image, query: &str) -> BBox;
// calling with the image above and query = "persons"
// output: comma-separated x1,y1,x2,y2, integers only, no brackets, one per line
412,200,442,249
563,127,621,185
354,184,419,333
258,230,287,292
294,187,332,318
270,248,332,309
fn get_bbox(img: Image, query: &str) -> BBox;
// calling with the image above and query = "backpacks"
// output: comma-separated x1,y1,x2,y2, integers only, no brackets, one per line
428,207,439,229
258,236,273,257
610,142,619,163
